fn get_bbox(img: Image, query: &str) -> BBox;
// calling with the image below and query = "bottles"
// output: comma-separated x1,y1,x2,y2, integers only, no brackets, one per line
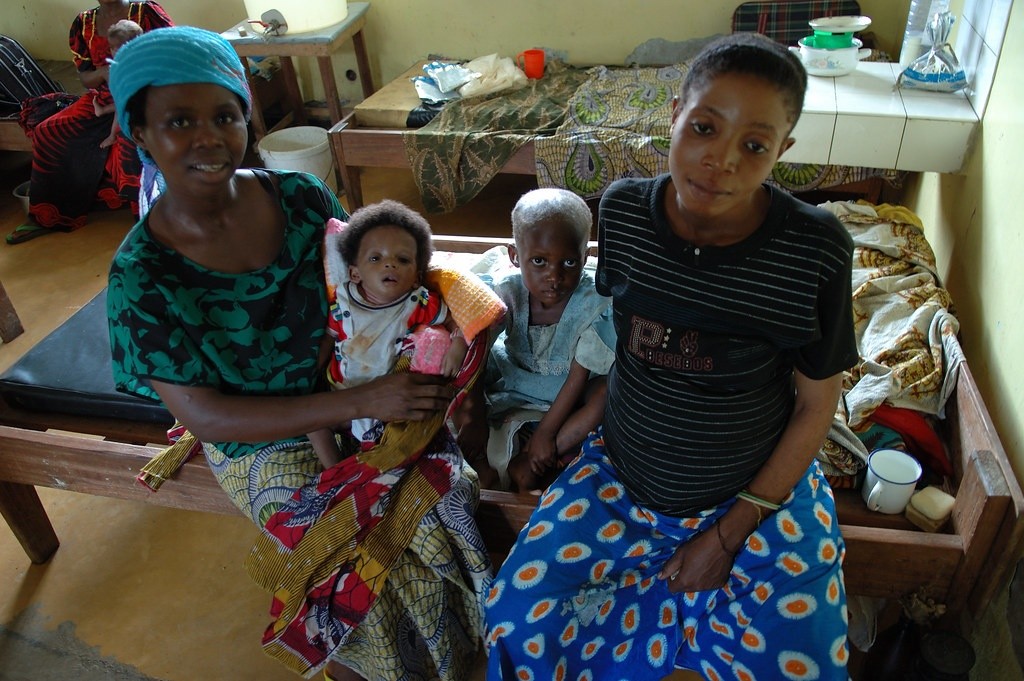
898,0,949,71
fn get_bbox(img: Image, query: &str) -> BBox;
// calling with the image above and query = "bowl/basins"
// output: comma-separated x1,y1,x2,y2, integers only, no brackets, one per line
13,180,32,216
787,37,871,77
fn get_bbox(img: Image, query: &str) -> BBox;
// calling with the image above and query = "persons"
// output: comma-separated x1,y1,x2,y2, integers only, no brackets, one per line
107,26,494,680
306,197,468,471
8,0,176,243
451,184,618,495
484,29,861,680
93,19,143,148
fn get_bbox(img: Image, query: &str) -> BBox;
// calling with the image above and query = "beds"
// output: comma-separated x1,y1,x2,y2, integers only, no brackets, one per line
0,199,1024,638
0,59,293,153
324,56,906,214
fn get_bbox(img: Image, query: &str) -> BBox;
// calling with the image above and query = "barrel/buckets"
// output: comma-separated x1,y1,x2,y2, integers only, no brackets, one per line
258,127,338,200
242,1,348,34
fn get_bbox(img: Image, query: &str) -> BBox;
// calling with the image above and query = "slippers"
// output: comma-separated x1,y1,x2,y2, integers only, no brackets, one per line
6,213,61,244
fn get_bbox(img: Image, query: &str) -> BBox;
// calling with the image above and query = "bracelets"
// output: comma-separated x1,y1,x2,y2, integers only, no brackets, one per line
734,485,783,528
714,517,741,559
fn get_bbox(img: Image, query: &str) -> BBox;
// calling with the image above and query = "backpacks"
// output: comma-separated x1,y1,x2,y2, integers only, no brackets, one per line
18,92,82,138
0,35,66,120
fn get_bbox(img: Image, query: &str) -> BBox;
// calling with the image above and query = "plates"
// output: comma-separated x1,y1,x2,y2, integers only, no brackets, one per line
808,15,872,33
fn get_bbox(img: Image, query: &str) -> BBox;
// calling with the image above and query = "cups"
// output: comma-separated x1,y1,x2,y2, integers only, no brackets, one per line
861,449,923,515
517,49,545,80
810,31,855,49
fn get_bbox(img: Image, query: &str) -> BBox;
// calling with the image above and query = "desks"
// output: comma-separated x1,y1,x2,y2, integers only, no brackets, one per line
219,2,376,147
777,61,979,174
